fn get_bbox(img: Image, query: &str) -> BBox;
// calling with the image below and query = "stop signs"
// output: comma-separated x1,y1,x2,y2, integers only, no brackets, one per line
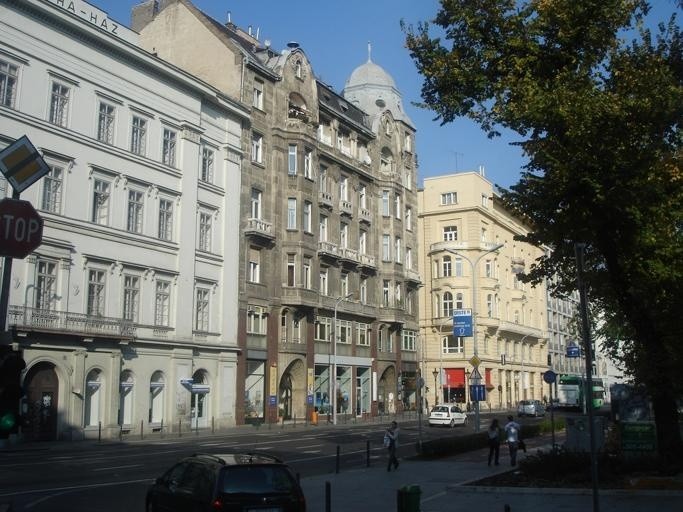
0,197,43,260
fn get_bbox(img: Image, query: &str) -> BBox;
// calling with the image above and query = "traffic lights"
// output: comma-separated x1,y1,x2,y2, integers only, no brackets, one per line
0,344,27,438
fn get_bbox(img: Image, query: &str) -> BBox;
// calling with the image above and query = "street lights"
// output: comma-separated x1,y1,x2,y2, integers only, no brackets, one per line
432,368,438,404
465,370,471,412
427,243,505,434
520,332,535,399
333,292,355,425
438,316,454,404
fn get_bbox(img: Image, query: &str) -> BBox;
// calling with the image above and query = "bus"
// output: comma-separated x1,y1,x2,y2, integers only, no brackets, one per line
557,375,606,411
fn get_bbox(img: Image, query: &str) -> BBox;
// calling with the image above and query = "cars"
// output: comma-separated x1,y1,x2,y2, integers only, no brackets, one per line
429,404,469,428
518,399,546,416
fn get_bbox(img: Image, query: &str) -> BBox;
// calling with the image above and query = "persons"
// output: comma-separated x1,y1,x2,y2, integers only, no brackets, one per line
485,419,500,466
325,410,333,425
384,419,400,472
504,415,521,466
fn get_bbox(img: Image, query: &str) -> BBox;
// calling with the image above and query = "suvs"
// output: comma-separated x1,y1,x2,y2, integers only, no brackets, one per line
146,450,308,512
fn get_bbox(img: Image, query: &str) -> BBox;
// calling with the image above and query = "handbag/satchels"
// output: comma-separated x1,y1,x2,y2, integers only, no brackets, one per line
384,437,390,447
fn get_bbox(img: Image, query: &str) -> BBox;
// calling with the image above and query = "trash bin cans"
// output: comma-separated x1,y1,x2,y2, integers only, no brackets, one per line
397,484,423,512
312,412,318,425
